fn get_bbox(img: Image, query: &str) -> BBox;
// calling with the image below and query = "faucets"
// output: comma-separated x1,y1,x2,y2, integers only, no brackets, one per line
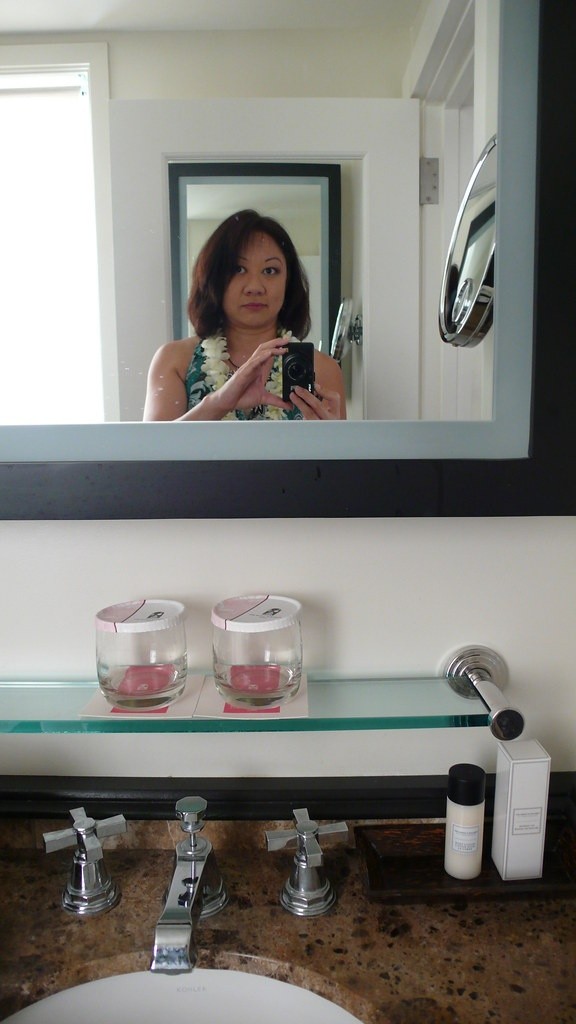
151,795,229,975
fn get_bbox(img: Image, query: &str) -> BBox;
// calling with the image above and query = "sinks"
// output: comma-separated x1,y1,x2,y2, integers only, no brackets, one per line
1,950,387,1024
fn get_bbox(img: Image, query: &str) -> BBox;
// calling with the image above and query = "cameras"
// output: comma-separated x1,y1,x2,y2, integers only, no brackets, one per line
281,342,315,402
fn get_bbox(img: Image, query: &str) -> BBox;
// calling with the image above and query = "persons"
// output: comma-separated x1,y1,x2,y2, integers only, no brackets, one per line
144,210,345,420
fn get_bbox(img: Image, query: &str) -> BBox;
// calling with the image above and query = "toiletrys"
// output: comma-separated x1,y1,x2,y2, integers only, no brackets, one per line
444,763,486,880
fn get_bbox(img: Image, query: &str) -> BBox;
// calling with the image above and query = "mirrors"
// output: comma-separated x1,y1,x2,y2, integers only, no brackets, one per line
168,163,342,369
0,0,576,521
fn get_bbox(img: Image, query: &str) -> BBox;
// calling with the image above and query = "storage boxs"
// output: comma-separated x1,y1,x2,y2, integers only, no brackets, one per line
491,739,551,881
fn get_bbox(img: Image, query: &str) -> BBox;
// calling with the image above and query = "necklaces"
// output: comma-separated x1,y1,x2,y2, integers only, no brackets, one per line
225,352,241,370
202,329,302,421
